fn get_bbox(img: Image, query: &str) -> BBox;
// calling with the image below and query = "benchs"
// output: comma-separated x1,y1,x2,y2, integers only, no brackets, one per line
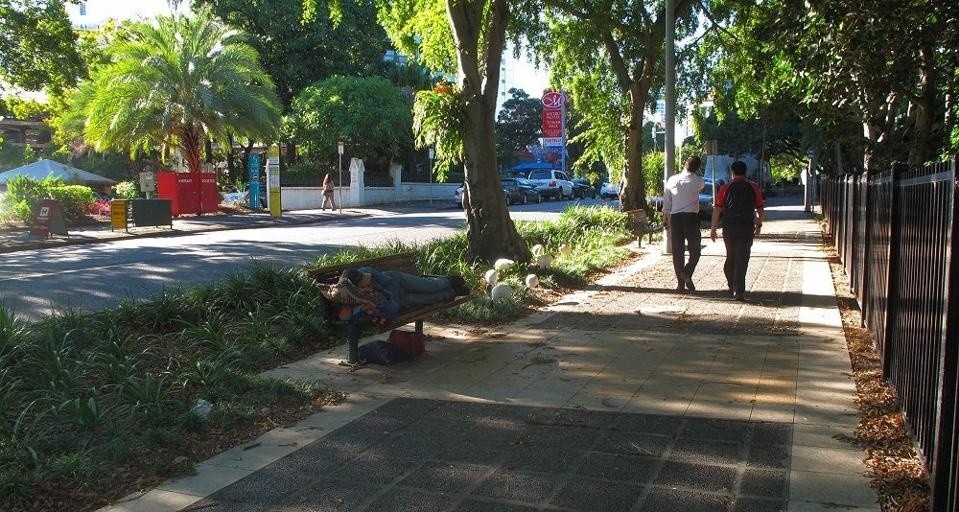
311,252,473,367
626,209,665,248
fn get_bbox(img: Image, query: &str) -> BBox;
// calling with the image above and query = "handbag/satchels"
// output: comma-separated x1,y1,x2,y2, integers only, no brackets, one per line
357,330,426,367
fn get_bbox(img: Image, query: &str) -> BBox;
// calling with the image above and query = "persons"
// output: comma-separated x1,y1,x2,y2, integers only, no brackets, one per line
321,172,340,212
661,154,706,293
339,266,471,321
709,159,766,301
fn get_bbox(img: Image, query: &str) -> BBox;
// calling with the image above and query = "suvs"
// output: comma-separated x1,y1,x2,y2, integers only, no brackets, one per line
529,168,575,200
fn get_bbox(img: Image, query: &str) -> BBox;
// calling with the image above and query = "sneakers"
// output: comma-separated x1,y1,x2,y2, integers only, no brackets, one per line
447,276,471,297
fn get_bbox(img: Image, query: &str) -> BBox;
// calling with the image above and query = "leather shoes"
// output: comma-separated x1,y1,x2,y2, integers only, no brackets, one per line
727,289,748,301
676,270,695,292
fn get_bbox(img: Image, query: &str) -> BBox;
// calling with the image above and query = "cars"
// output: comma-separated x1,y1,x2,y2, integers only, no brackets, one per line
455,182,511,207
699,182,721,217
500,177,542,204
570,178,596,199
602,186,619,200
